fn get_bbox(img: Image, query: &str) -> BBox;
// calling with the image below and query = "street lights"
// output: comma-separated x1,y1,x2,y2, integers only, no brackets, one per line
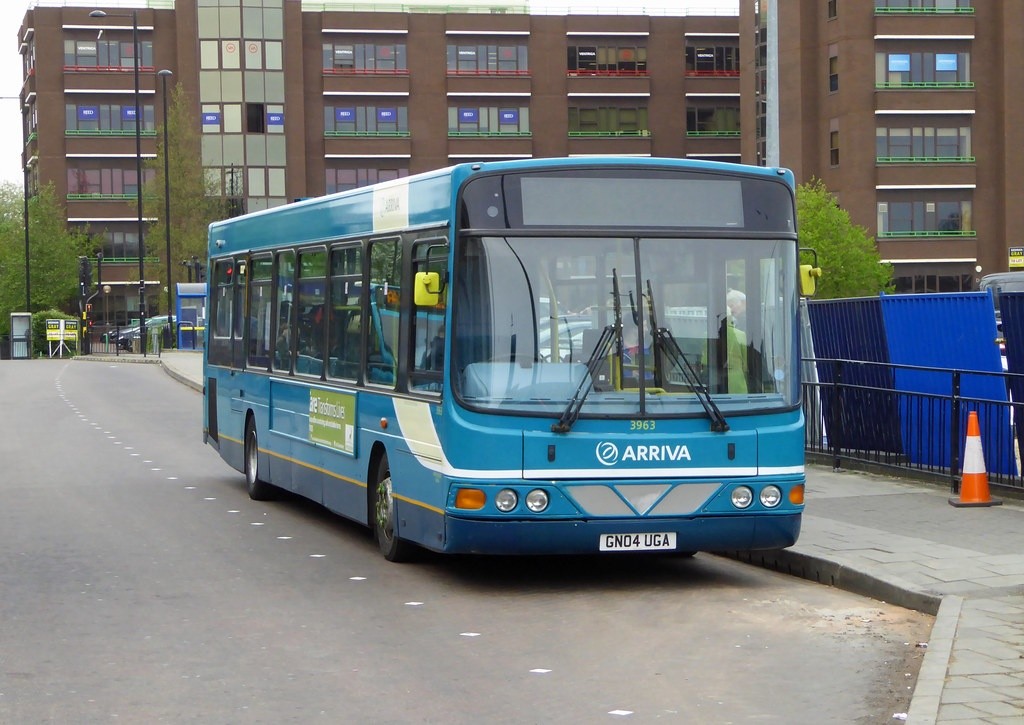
90,9,148,353
103,285,112,353
159,67,175,346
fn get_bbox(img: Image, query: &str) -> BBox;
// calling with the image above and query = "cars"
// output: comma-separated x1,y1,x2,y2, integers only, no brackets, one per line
100,315,176,352
536,296,628,370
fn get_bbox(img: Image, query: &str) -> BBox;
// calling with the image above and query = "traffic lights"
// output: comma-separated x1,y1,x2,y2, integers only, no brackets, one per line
86,304,92,312
86,320,93,328
312,304,336,324
217,259,234,284
79,260,89,285
83,312,90,321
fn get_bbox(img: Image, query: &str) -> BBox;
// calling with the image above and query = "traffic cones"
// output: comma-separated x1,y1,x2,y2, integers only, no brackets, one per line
948,410,1003,507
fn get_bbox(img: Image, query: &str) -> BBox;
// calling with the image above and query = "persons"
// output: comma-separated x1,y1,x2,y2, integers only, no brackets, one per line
276,326,301,371
616,311,654,379
701,287,767,393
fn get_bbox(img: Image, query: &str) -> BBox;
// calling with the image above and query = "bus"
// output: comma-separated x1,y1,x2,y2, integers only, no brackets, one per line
201,158,822,565
